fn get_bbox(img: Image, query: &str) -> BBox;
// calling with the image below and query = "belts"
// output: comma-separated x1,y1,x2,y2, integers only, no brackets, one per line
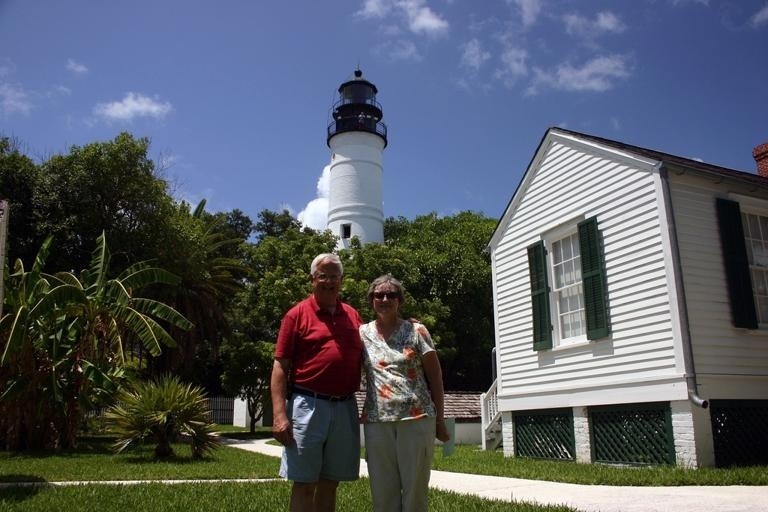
292,386,354,402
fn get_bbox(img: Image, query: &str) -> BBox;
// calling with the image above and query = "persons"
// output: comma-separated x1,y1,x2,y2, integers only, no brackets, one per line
269,252,421,511
358,273,452,512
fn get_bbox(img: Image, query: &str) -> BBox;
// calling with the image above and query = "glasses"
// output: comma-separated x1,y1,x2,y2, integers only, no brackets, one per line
375,292,398,300
313,272,337,283
